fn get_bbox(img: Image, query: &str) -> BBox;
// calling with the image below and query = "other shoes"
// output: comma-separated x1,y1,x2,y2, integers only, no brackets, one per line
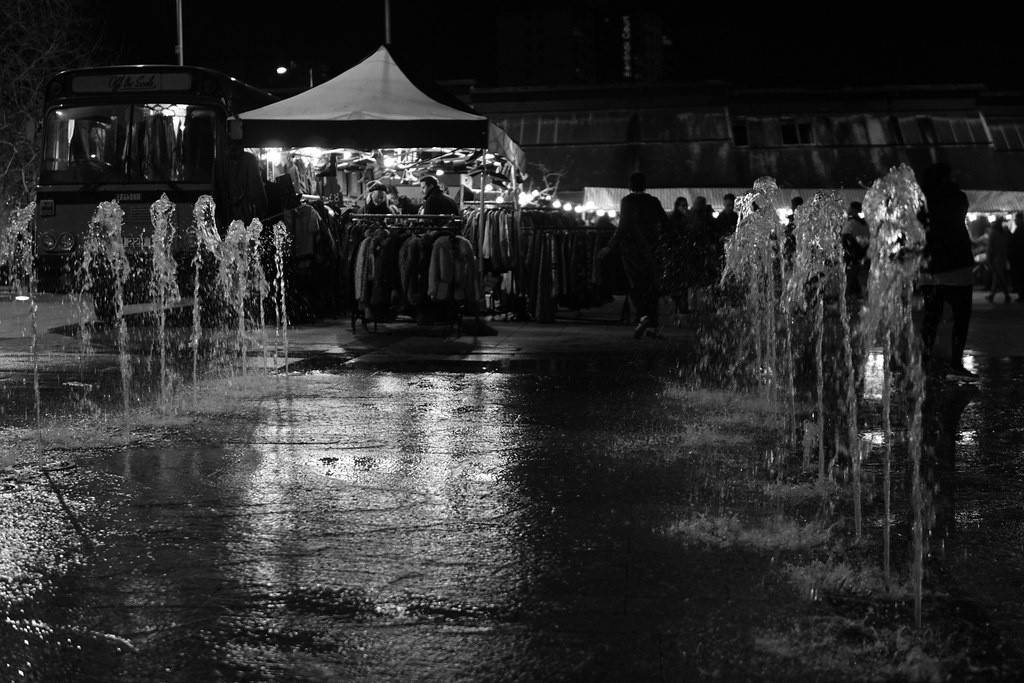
946,364,978,382
1015,299,1024,303
1005,296,1010,303
985,296,993,303
647,326,656,337
634,318,649,338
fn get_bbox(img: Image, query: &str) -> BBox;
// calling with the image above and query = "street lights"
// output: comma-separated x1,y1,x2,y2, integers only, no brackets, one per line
275,66,315,91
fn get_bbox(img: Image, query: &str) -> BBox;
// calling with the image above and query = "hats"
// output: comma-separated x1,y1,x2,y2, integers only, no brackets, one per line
369,184,386,191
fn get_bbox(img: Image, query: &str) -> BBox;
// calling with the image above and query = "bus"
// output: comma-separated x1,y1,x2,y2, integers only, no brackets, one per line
29,62,294,300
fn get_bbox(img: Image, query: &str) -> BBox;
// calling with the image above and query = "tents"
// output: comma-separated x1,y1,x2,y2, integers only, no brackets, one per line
224,44,526,286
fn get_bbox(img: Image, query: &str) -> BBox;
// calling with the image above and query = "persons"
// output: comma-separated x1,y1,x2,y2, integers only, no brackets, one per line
598,165,1024,374
360,176,459,219
